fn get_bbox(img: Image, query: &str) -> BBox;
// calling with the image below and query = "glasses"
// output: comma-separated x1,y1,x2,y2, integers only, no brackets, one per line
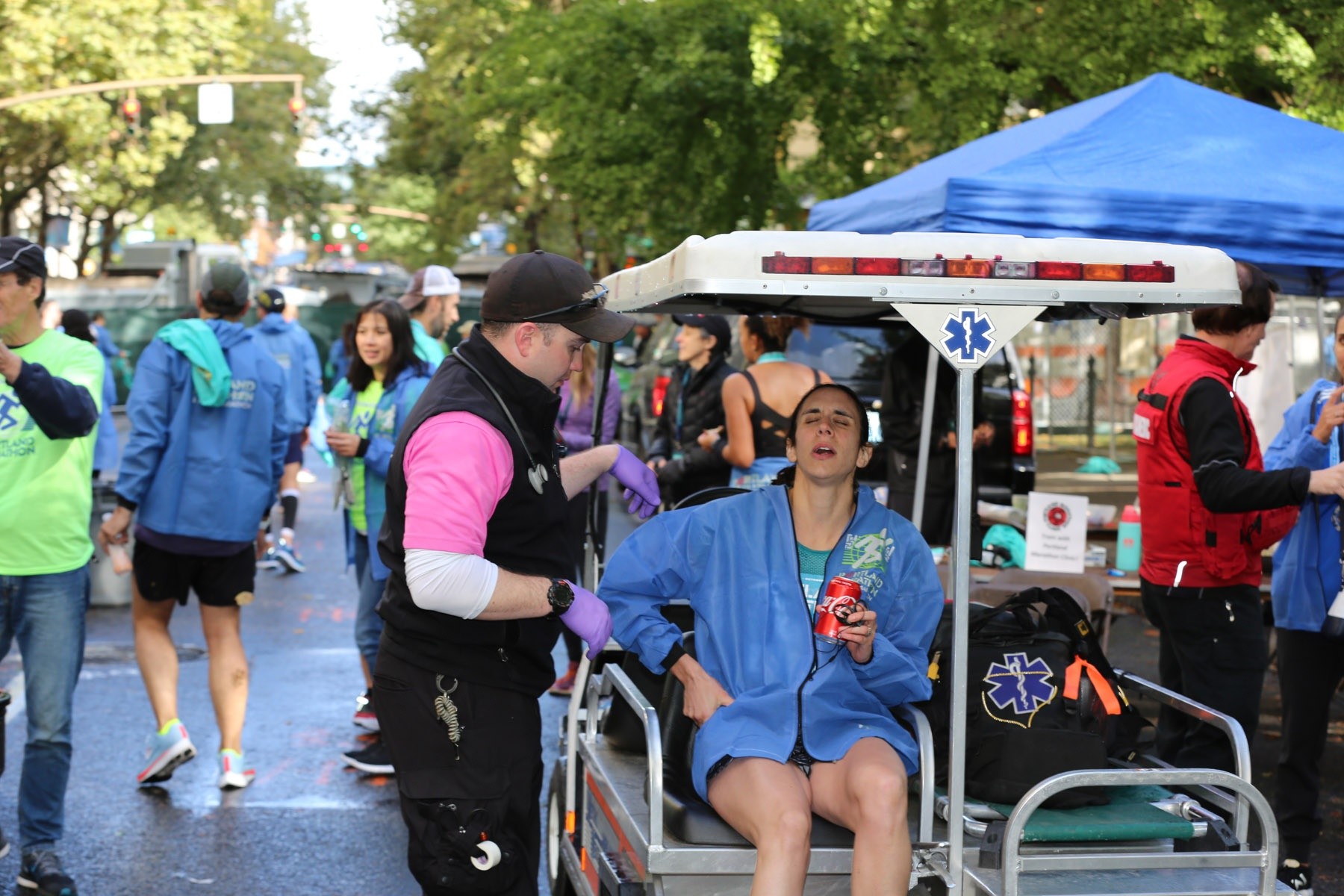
523,283,609,320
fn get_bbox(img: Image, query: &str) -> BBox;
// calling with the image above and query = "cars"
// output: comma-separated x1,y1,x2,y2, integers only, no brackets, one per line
65,238,509,342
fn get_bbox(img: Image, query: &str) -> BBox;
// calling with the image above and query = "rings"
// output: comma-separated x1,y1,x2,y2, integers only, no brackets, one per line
868,626,872,635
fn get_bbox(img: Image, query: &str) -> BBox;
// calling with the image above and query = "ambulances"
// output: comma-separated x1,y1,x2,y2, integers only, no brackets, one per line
537,233,1279,896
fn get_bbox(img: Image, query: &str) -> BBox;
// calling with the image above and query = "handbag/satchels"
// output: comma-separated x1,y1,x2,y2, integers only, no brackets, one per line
917,586,1141,809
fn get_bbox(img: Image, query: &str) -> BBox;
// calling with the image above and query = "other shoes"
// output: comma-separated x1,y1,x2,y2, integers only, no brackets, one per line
137,723,196,784
340,743,396,774
1276,865,1314,896
0,836,10,858
256,551,280,568
16,850,77,896
275,541,306,573
216,749,255,789
354,703,380,732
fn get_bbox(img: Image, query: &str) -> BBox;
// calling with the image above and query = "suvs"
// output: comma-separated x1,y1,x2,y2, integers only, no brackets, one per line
726,314,1037,507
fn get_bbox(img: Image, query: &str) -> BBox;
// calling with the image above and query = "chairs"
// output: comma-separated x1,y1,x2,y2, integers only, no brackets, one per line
599,605,854,847
972,569,1114,655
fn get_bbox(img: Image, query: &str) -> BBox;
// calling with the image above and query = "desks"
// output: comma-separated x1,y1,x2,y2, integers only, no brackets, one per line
970,563,1272,656
1035,471,1138,492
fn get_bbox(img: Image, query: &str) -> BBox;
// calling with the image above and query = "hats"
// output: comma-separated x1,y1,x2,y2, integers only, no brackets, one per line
200,262,249,307
254,288,285,310
0,236,45,273
671,312,731,343
398,264,461,310
479,249,636,343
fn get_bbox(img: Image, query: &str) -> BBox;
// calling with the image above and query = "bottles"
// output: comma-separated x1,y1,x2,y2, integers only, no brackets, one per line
1115,504,1142,572
331,398,351,468
101,512,134,576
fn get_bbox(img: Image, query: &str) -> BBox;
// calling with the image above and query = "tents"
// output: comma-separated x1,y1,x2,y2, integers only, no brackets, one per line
806,71,1344,530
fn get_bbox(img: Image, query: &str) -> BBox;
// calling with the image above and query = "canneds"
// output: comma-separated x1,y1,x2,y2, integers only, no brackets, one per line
814,576,862,645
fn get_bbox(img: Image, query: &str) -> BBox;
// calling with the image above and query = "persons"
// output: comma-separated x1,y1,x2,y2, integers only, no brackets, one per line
371,249,636,896
593,384,944,896
0,236,107,896
61,263,831,789
1131,261,1344,896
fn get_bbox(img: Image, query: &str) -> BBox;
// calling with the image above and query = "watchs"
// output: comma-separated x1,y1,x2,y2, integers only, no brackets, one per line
547,577,575,616
